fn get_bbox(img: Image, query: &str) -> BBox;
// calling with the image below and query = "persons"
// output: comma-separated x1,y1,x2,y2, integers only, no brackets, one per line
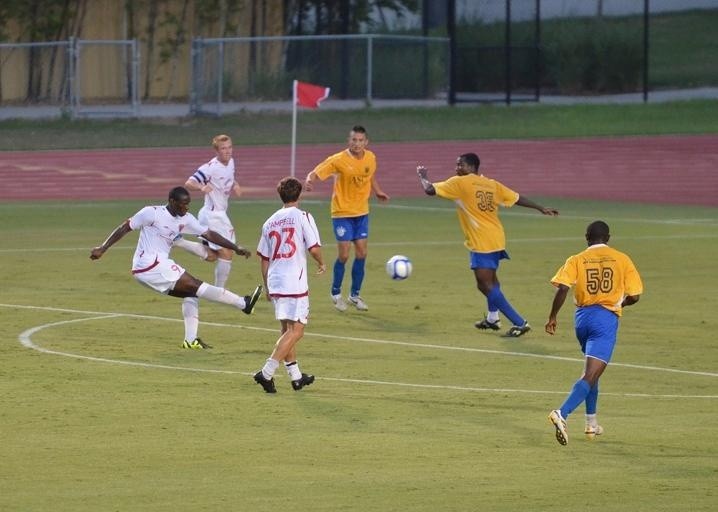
304,127,391,314
86,185,262,351
415,152,562,338
172,135,247,291
544,221,645,447
255,176,325,393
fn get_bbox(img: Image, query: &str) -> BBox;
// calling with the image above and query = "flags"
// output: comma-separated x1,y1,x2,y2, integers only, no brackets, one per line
293,80,331,107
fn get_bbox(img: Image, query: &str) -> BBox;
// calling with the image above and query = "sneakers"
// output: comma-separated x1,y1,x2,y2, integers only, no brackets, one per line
183,338,212,349
292,374,314,391
331,289,347,312
348,293,368,311
254,371,276,392
242,284,263,314
506,320,530,337
548,410,568,445
476,312,500,329
584,424,603,434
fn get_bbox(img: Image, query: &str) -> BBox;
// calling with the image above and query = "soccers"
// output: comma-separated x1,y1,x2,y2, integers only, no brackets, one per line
386,254,413,282
